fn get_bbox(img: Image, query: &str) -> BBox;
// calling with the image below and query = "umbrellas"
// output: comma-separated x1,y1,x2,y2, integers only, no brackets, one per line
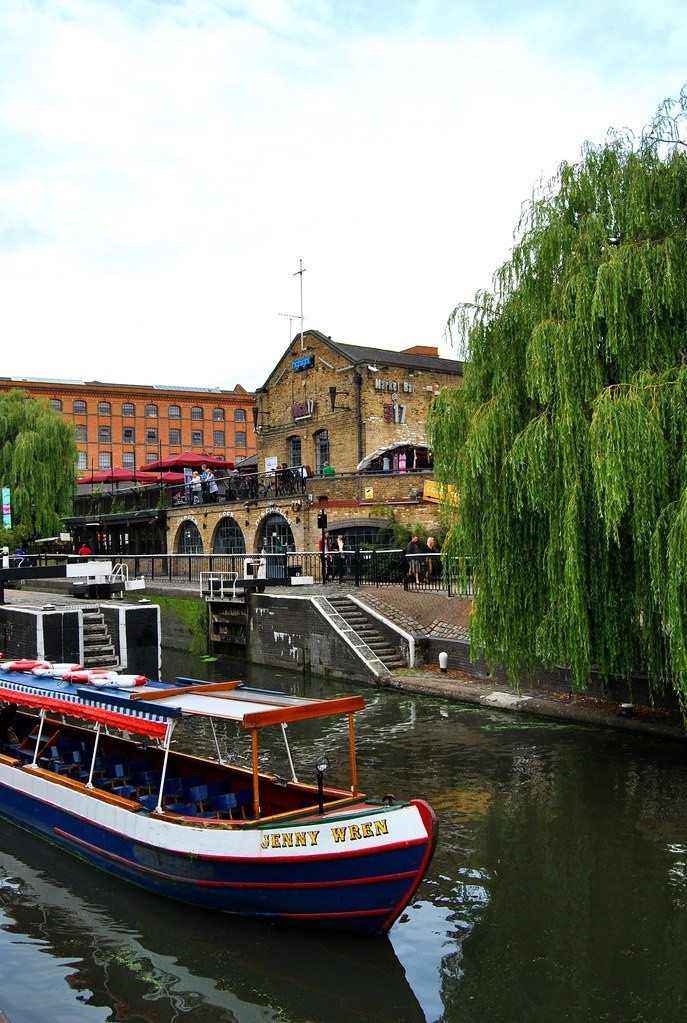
77,465,159,491
140,450,234,471
156,470,184,484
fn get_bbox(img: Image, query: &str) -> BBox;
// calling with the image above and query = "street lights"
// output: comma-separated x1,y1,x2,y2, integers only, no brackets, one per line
314,491,330,584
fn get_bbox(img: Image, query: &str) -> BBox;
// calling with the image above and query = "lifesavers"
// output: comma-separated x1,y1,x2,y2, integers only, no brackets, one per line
62,669,119,684
31,662,84,678
1,660,52,672
90,674,148,688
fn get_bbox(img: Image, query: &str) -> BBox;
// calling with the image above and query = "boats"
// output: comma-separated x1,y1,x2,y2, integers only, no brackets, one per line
0,656,439,948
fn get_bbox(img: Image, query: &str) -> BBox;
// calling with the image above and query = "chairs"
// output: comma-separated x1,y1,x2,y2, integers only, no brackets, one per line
12,731,256,820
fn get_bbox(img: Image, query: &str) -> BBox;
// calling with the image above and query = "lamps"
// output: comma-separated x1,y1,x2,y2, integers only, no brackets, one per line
330,386,350,411
203,523,206,529
411,492,423,502
166,526,170,531
251,406,270,427
245,520,249,526
405,369,414,373
255,388,268,394
296,517,300,523
381,366,389,370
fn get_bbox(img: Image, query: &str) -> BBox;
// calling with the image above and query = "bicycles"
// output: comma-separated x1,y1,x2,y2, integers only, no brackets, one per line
236,468,306,497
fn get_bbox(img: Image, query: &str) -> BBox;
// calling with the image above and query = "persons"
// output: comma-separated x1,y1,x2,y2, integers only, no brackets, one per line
321,460,335,477
332,532,346,583
319,531,332,585
189,463,219,505
78,543,92,554
15,545,26,559
228,464,314,500
422,537,437,582
403,535,422,585
